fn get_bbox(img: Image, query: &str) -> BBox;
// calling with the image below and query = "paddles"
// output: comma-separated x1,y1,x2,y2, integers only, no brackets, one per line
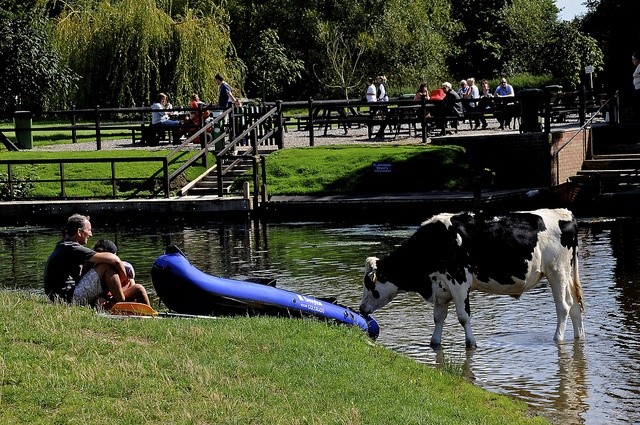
109,302,223,319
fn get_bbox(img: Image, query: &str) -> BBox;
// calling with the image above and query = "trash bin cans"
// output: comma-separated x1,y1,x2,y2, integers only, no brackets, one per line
401,94,416,106
14,111,33,149
519,89,544,132
615,89,631,123
548,85,564,114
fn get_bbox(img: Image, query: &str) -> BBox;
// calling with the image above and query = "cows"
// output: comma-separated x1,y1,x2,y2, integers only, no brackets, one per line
359,208,588,350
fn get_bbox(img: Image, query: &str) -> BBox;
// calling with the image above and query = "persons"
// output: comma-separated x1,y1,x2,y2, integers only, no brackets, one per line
364,77,377,102
215,74,236,108
150,92,182,145
190,94,211,141
474,80,490,129
467,78,480,109
234,99,248,147
441,82,464,120
92,239,151,307
377,76,388,102
44,214,127,304
458,80,469,97
628,54,640,94
494,77,515,129
414,84,429,100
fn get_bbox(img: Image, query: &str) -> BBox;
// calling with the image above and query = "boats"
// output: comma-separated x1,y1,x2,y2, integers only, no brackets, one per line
150,252,380,342
